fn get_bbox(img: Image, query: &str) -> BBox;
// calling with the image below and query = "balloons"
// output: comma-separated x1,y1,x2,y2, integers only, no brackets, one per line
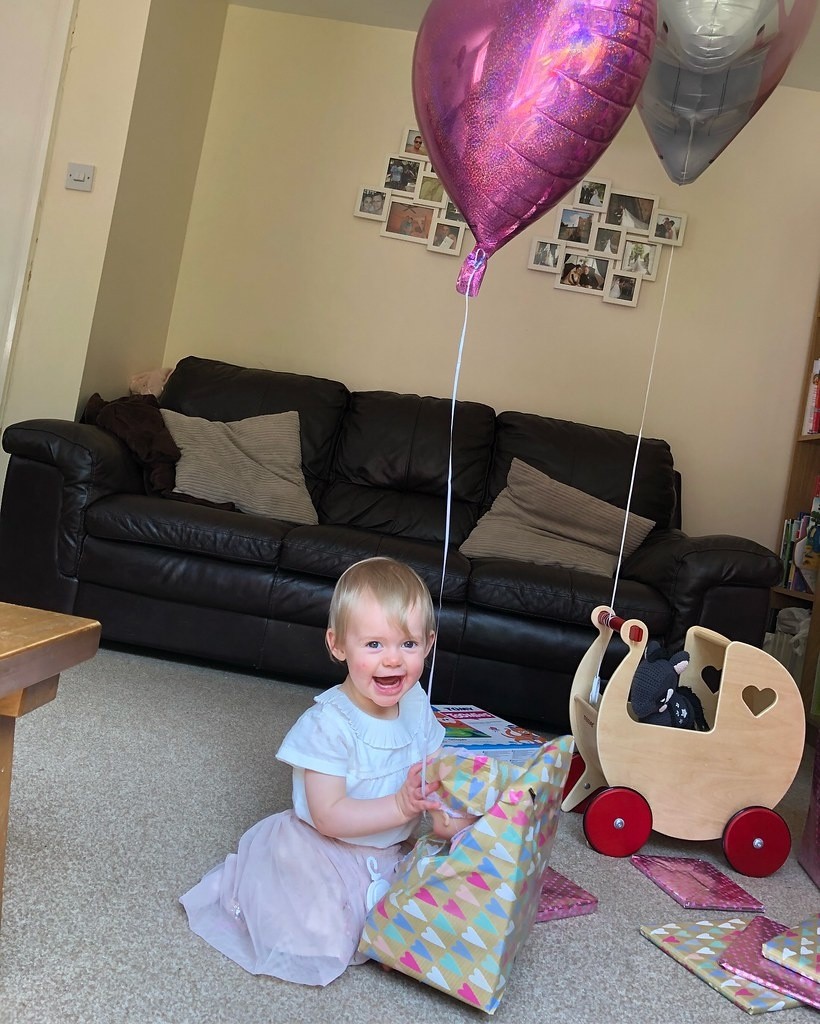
412,0,657,297
635,0,820,187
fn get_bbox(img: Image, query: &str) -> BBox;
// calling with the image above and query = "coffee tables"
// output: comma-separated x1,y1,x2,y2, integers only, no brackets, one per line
1,600,103,926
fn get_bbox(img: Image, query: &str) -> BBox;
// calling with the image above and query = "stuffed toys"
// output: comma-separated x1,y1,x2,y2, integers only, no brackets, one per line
630,640,713,732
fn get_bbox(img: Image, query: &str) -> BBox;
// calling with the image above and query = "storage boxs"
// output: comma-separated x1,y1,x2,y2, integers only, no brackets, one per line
429,704,549,768
357,734,577,1014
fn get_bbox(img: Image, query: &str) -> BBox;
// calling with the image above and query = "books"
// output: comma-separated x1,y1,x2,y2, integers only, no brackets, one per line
777,496,820,595
762,631,820,718
801,359,820,437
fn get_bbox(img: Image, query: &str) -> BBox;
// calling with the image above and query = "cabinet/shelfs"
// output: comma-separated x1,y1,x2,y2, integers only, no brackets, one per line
759,274,820,751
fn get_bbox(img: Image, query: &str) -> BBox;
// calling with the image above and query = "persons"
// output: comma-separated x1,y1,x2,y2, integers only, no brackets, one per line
530,177,678,305
179,556,483,989
358,129,469,254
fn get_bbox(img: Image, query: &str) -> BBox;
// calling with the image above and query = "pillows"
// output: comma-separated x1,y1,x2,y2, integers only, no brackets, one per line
460,456,656,580
159,409,319,525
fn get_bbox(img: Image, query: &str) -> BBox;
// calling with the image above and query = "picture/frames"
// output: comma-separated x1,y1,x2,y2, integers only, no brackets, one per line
648,209,687,247
527,237,567,274
379,154,425,198
412,171,449,209
379,196,439,244
572,176,612,213
399,127,431,162
553,250,615,295
427,217,466,256
615,236,663,282
588,221,627,260
424,162,434,172
354,184,392,221
440,198,471,229
553,203,599,250
601,189,660,236
602,269,643,308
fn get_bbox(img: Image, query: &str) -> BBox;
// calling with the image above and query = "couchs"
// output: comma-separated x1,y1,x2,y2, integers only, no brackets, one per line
0,355,782,736
